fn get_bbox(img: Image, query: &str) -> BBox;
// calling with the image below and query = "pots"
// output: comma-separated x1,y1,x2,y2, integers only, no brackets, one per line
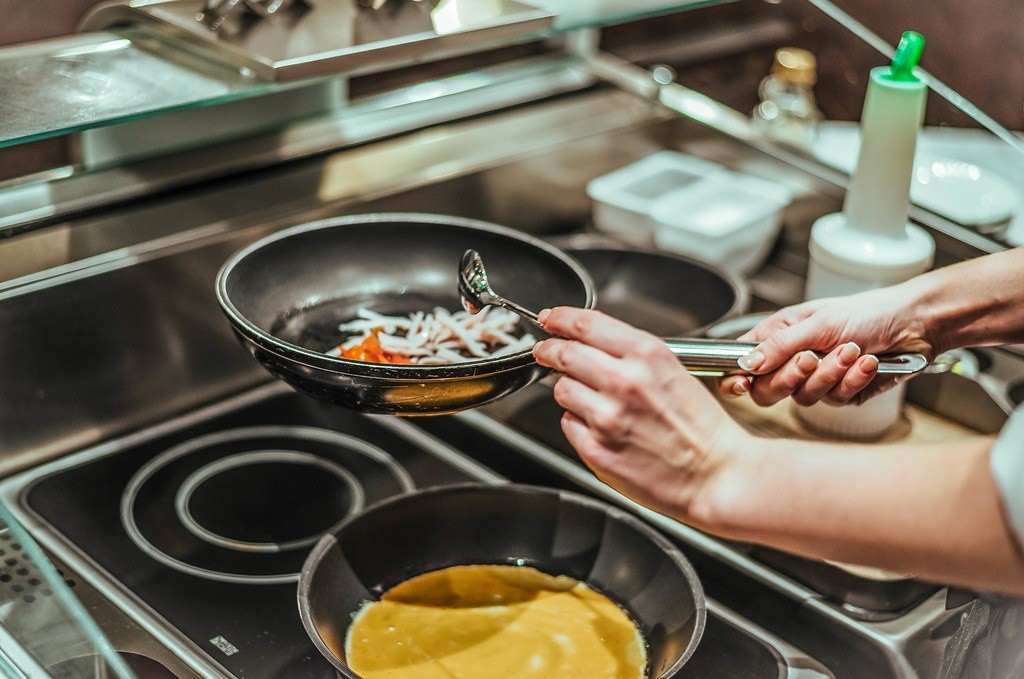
565,244,778,336
214,212,929,417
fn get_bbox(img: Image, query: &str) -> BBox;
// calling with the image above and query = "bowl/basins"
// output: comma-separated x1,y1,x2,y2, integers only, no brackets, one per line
298,483,706,679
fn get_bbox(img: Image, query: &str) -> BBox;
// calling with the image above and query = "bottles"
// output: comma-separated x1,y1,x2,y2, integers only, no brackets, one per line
754,46,820,157
795,32,935,437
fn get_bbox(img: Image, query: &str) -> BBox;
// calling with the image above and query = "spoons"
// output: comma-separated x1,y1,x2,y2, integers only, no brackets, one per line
456,249,561,339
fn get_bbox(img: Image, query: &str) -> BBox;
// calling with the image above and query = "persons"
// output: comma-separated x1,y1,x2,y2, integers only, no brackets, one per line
532,251,1024,603
